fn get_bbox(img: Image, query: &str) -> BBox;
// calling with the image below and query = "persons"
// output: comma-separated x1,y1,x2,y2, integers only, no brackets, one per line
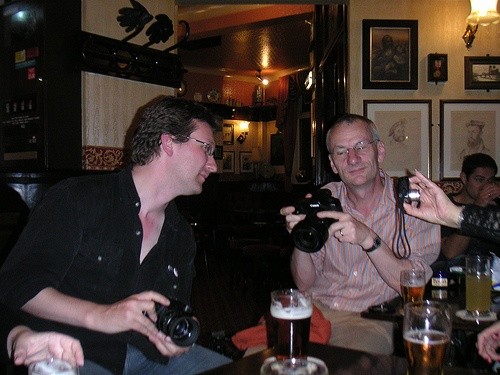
0,94,234,375
243,113,441,357
403,153,500,362
0,180,84,375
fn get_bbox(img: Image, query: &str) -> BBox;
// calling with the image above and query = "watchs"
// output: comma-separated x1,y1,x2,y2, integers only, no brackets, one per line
362,234,381,251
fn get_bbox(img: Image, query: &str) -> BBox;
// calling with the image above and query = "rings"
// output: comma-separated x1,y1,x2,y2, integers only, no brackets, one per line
340,230,344,237
488,194,491,197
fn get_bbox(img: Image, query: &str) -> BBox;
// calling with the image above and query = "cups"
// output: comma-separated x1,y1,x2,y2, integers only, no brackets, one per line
399,270,425,317
465,252,493,317
404,300,452,375
270,288,314,375
27,351,79,375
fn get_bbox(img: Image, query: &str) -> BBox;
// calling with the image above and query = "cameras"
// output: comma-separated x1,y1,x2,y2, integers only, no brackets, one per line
290,191,344,252
142,295,201,347
397,176,421,212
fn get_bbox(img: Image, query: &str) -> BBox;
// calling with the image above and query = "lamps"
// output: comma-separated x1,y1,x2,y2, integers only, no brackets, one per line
256,69,269,86
236,121,249,144
462,0,500,48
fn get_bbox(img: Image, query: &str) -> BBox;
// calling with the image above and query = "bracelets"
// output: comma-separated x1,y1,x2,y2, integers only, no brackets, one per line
455,206,465,228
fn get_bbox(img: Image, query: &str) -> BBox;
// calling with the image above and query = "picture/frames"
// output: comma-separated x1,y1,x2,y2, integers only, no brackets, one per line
362,19,418,90
363,99,432,181
213,145,223,160
439,98,500,182
222,151,235,174
239,151,252,174
222,123,234,145
464,55,500,90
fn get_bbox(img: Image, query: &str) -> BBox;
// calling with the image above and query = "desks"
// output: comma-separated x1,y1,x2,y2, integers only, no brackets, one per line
360,287,500,331
195,342,496,375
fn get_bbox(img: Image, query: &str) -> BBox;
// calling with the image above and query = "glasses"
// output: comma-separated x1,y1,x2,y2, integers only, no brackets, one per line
330,138,379,158
159,134,214,157
